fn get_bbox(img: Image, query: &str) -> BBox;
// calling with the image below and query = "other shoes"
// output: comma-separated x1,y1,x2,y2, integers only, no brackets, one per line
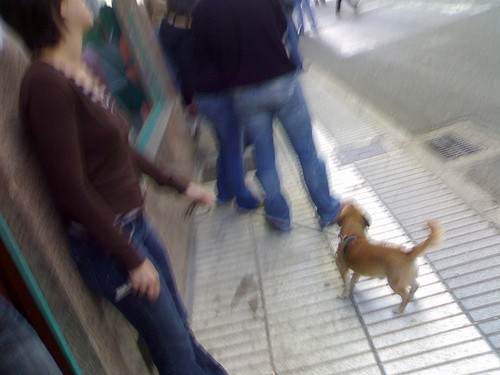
237,196,265,214
325,204,341,226
216,197,231,205
264,215,291,231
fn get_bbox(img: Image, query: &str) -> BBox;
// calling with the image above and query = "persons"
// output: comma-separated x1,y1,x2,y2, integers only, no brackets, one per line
1,0,233,375
334,0,343,18
279,0,305,72
296,0,318,37
314,0,327,8
192,0,345,232
156,0,264,214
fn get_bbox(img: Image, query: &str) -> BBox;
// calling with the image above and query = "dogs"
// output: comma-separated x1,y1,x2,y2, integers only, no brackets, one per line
335,202,443,316
